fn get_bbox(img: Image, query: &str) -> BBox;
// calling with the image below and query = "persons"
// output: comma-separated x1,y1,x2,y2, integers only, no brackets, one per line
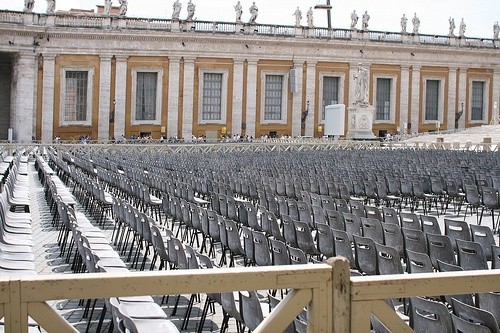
292,6,303,27
448,17,456,35
171,0,182,20
493,21,500,38
28,129,303,143
400,14,408,32
459,18,467,37
249,2,259,23
185,0,197,21
307,7,314,28
362,11,371,29
412,12,421,33
350,10,358,29
233,1,243,22
119,0,128,15
104,0,113,15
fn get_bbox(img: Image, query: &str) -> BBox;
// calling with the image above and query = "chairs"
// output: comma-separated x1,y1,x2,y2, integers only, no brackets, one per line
0,139,500,333
